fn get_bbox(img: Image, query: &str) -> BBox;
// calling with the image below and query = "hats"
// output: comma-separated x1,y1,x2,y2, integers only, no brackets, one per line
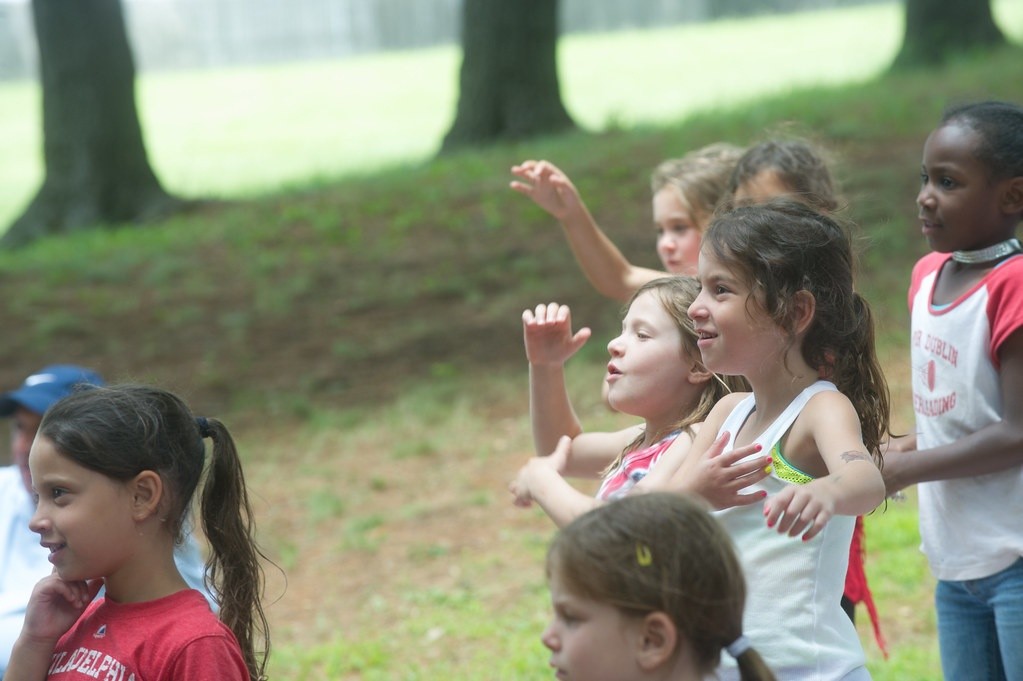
0,363,103,418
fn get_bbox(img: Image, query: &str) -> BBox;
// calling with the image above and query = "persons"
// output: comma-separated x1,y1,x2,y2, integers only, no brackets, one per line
0,361,276,681
511,134,901,681
544,491,777,681
870,101,1023,681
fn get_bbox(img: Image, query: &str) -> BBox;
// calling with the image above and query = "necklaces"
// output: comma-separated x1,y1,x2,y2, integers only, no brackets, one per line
953,239,1023,264
